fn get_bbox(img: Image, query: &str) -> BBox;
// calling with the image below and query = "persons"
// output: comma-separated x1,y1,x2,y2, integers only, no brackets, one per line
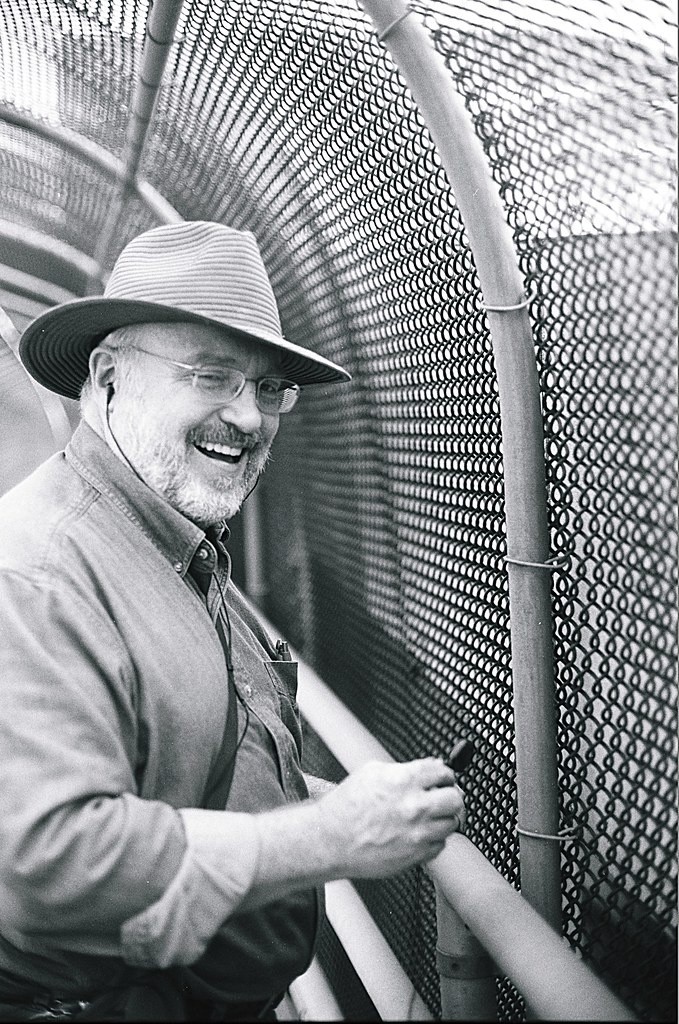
0,222,465,1024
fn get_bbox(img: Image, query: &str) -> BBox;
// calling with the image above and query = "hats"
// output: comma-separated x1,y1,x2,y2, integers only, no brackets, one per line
18,221,351,401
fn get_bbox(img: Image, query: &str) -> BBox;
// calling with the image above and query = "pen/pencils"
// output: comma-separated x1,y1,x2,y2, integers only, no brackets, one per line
275,638,293,661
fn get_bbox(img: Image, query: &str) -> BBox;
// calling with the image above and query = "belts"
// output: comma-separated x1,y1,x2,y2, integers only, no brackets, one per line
0,978,285,1024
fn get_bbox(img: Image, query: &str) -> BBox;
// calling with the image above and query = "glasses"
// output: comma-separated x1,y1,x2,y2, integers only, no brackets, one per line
110,346,300,414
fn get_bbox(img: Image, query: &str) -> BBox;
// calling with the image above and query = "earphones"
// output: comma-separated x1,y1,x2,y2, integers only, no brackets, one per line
105,383,115,405
